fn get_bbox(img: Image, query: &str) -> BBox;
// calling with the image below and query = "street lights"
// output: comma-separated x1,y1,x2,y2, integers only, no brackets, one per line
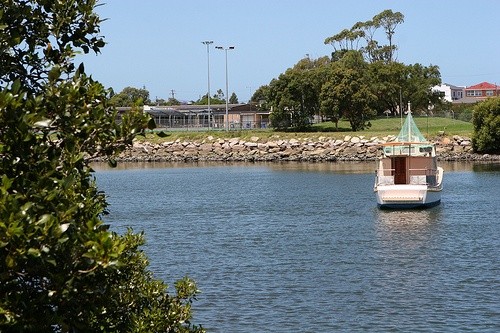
202,41,213,132
215,46,234,133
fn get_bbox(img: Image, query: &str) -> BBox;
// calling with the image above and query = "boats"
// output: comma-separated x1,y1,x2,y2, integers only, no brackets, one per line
374,102,445,210
